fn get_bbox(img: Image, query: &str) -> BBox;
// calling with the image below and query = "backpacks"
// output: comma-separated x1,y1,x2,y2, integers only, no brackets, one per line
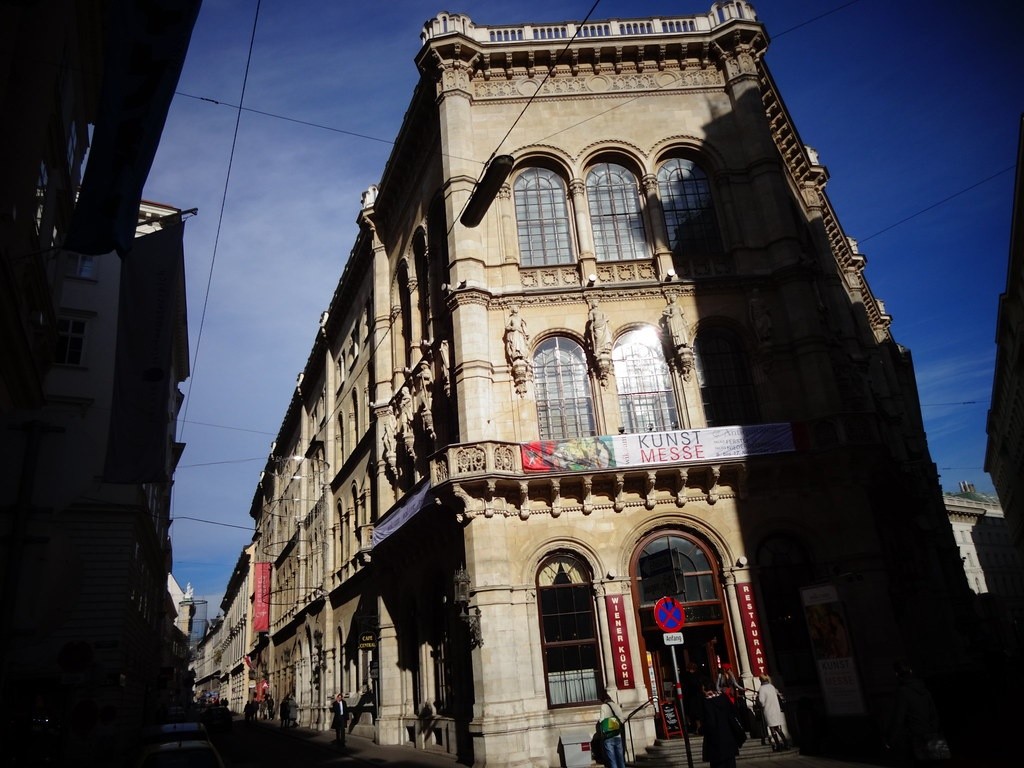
729,717,747,748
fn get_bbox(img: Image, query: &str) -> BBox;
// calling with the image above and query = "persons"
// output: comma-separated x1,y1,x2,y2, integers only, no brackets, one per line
808,606,847,659
243,696,274,721
882,656,940,768
758,674,790,752
716,664,746,700
695,680,747,768
677,663,702,734
279,696,299,729
330,693,349,746
213,697,229,707
597,691,625,768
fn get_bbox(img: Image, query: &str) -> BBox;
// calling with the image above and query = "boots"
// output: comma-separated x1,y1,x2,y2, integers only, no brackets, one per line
773,735,783,752
780,733,792,750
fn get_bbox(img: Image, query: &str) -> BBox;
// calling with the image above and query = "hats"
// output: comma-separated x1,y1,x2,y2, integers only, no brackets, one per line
722,664,732,669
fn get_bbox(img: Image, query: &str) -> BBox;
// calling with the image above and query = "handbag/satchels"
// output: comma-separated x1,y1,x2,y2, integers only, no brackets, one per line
599,714,624,739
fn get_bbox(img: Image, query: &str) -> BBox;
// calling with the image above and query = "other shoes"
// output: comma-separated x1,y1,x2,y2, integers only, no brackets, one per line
342,743,346,747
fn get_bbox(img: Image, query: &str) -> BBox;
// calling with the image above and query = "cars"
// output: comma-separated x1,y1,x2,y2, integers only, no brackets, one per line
138,740,224,768
199,707,233,729
145,722,209,746
